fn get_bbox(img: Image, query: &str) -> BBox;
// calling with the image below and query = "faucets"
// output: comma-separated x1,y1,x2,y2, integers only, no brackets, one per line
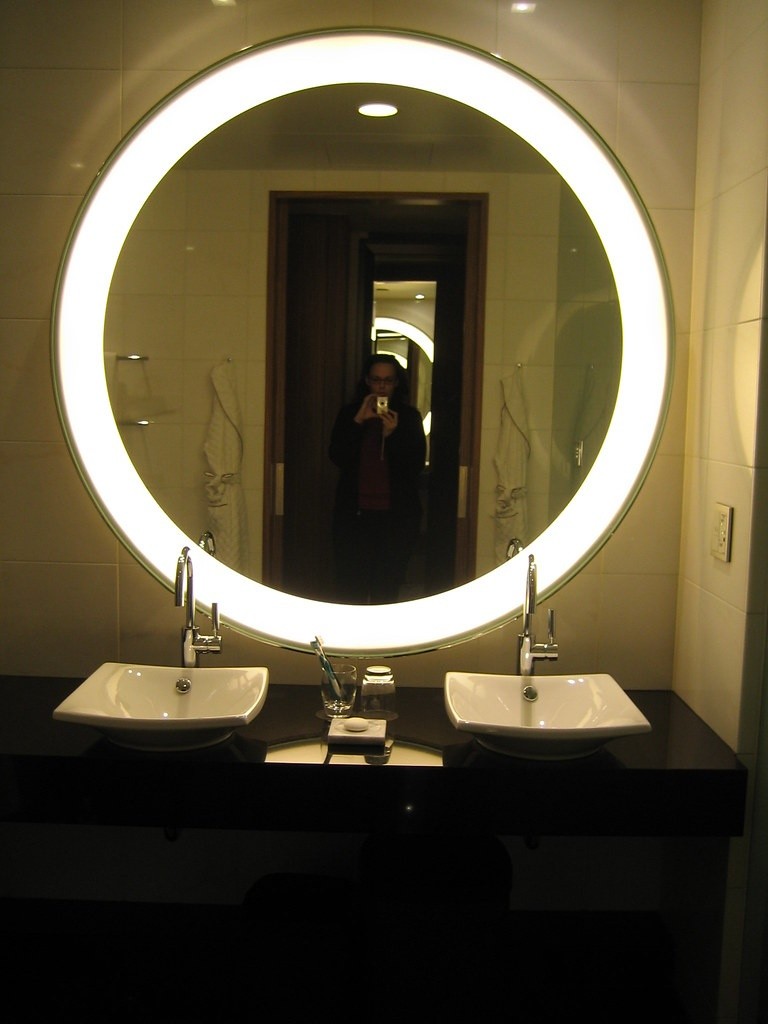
173,547,223,668
506,538,523,558
518,553,558,675
197,530,215,556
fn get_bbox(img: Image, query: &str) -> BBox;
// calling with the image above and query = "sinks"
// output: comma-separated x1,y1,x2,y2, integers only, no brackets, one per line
53,662,269,751
444,671,653,761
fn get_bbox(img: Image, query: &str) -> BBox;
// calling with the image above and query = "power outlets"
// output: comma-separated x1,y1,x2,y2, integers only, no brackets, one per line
711,501,732,562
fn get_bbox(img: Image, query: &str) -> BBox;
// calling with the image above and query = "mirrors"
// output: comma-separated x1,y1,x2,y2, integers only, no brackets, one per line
48,27,678,662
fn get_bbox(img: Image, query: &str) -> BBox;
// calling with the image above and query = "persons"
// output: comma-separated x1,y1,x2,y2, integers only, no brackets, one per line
329,354,427,605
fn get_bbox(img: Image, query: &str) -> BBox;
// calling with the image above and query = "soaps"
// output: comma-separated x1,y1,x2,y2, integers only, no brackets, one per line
344,716,369,732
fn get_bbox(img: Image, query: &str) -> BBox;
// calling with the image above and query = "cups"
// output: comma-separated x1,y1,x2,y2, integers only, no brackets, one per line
321,664,357,718
361,666,397,720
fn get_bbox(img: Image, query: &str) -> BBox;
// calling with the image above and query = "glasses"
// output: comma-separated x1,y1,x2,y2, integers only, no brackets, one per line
369,376,397,384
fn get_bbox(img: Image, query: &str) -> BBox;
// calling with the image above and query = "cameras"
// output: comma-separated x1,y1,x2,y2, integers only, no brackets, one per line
376,397,388,414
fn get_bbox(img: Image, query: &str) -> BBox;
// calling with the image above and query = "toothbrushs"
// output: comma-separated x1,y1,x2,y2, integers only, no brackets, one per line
310,634,347,701
